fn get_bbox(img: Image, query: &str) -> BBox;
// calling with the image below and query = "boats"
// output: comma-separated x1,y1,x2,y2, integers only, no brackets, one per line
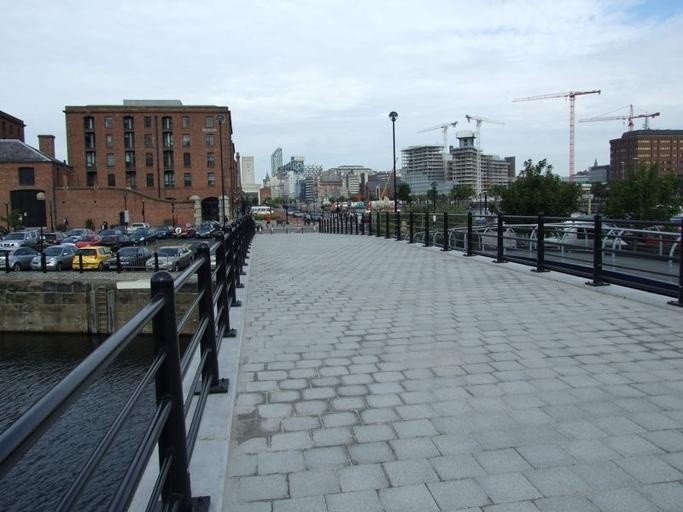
544,220,628,247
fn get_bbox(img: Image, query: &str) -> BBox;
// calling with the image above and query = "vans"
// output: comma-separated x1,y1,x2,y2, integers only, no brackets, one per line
249,206,275,216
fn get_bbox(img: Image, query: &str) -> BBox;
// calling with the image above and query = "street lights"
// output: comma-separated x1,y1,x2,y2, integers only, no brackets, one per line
36,192,46,271
389,112,398,239
216,114,225,234
431,182,438,235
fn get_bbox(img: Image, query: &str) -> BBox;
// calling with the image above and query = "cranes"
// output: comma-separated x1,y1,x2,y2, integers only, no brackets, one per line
417,121,457,146
465,114,504,150
578,104,660,132
512,91,601,184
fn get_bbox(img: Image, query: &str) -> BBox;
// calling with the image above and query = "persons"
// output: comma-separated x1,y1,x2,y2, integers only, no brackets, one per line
302,214,310,224
64,218,69,231
277,215,283,226
101,221,108,231
266,212,272,224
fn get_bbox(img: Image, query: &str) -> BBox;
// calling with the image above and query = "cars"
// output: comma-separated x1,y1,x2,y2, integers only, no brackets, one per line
281,205,321,221
0,221,216,271
332,202,349,208
